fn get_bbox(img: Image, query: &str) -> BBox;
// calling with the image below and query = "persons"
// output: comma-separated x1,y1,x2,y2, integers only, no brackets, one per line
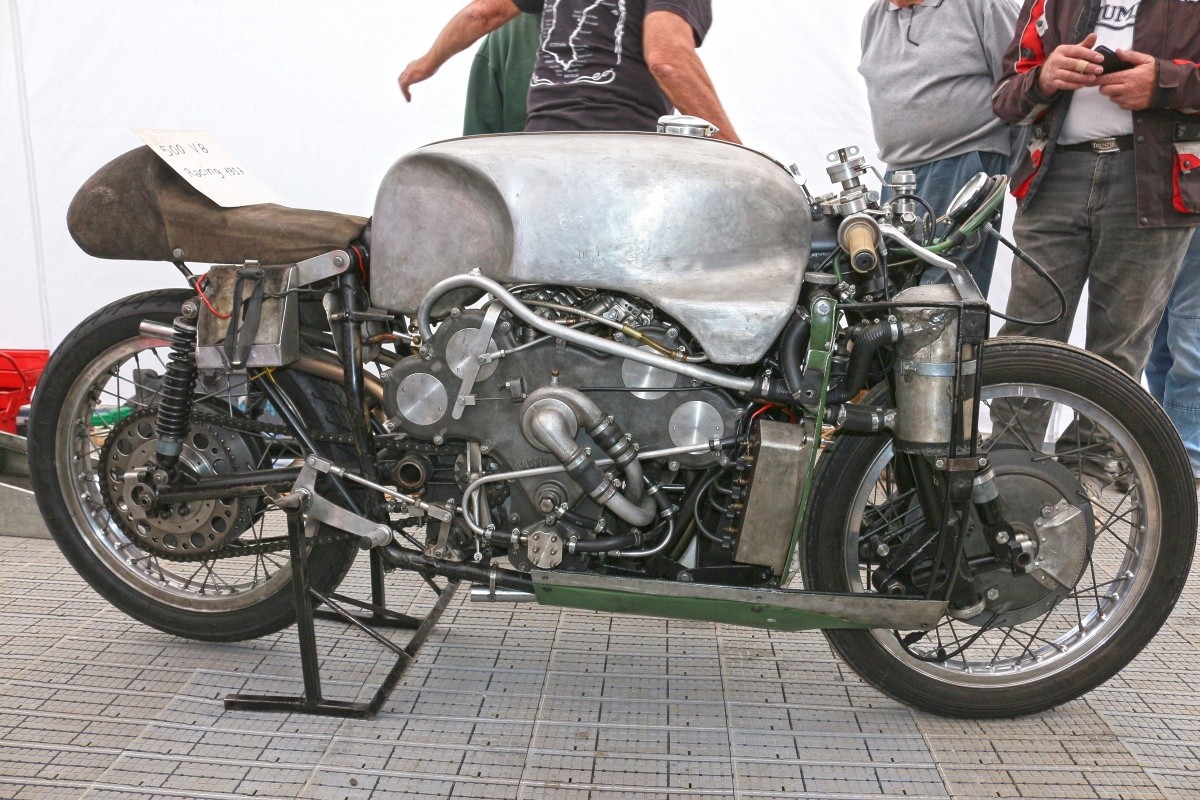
856,0,1200,514
396,0,745,150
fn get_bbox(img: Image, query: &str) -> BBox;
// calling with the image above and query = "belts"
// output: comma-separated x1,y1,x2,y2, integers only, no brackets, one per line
1054,134,1134,154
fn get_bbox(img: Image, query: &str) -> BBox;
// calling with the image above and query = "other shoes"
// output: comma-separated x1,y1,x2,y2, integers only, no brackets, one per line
1075,474,1103,526
1116,462,1131,492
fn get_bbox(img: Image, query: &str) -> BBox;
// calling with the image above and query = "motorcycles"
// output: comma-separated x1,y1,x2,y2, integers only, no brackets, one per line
21,100,1198,722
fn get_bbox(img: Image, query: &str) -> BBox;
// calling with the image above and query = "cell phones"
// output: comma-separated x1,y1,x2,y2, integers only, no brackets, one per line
1091,45,1134,77
